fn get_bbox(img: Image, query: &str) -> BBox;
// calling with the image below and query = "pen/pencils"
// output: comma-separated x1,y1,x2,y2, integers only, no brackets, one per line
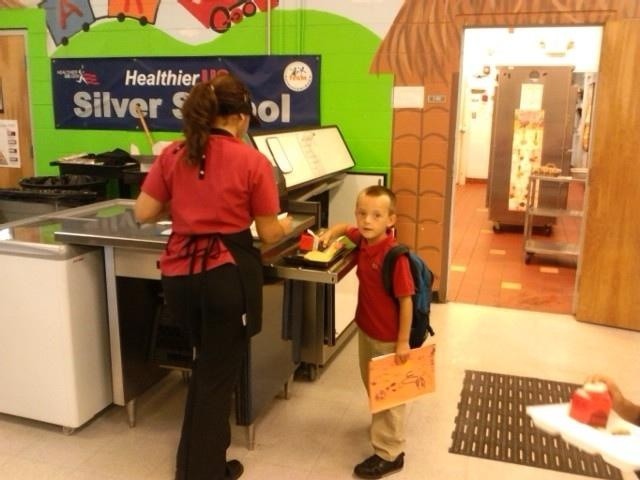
307,229,319,239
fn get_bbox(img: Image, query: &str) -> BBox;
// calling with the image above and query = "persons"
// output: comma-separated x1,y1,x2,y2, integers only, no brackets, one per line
317,185,418,478
131,74,293,480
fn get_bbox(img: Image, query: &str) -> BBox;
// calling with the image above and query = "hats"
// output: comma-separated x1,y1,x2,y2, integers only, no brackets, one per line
218,94,259,129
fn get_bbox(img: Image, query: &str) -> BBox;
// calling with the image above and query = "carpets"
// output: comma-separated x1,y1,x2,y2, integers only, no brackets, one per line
448,368,621,480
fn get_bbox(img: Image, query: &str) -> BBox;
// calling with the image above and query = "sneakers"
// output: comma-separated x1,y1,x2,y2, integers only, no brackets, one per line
214,460,244,478
354,452,404,479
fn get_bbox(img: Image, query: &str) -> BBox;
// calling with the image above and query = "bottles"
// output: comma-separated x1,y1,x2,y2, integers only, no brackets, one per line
568,381,614,430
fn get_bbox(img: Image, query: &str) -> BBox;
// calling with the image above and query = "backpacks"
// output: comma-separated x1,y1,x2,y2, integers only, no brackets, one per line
382,243,433,349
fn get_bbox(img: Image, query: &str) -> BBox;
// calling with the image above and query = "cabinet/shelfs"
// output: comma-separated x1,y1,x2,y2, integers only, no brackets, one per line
522,171,588,267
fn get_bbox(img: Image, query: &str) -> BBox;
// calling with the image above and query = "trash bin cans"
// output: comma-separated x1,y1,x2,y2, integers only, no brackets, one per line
0,175,109,224
